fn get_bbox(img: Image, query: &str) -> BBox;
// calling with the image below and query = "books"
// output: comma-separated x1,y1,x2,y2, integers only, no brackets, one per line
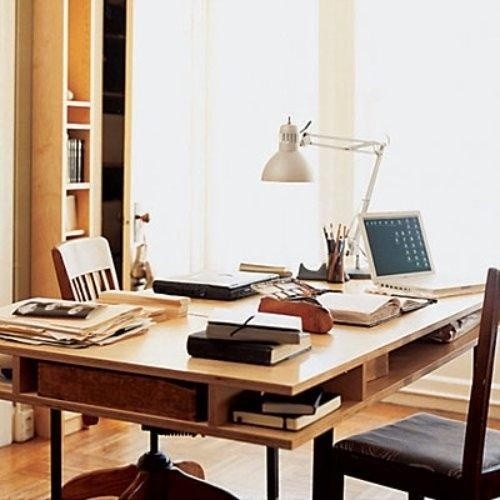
0,296,159,348
232,392,341,432
240,261,293,277
186,330,311,364
262,389,323,415
65,132,86,230
314,285,437,327
205,308,303,345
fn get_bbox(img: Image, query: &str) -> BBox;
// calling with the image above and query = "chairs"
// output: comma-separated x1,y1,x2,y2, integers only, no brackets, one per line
50,236,240,500
324,268,500,500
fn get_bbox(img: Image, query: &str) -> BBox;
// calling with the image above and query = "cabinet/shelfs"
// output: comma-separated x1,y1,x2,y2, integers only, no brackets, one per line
30,0,102,440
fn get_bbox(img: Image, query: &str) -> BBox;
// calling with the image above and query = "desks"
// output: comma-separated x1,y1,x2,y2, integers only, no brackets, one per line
1,273,485,500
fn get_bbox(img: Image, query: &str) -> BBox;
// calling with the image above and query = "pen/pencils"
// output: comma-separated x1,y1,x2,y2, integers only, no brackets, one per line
323,223,350,283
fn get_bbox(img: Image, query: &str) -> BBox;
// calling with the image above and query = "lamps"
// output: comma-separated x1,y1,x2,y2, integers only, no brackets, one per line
262,116,388,280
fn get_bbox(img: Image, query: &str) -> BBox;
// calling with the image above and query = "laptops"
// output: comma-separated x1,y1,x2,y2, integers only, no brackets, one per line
356,211,486,299
